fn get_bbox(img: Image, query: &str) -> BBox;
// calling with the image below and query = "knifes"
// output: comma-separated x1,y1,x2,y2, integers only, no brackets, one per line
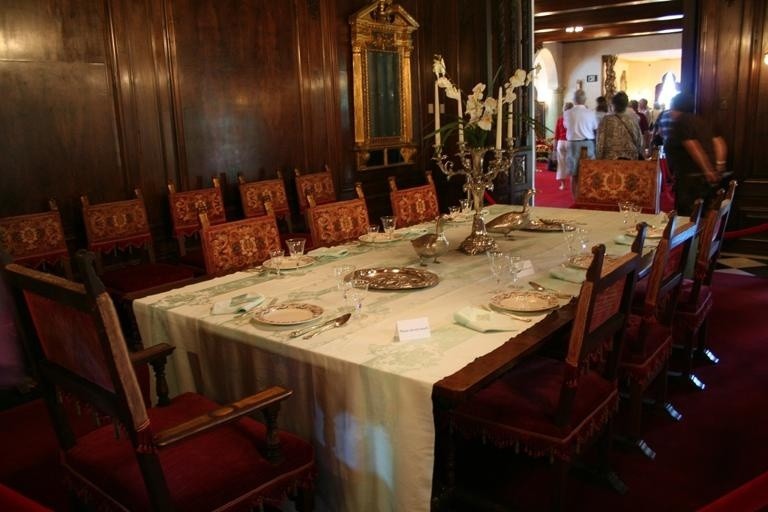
291,316,341,337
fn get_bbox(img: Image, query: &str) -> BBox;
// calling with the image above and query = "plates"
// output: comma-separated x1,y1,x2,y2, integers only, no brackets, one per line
439,209,489,226
262,255,314,271
252,304,324,326
343,267,440,290
489,290,560,311
359,232,402,243
566,255,619,270
625,225,667,238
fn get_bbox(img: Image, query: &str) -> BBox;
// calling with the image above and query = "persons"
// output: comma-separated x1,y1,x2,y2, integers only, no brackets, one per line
562,88,599,196
551,102,573,190
662,90,729,216
594,91,676,161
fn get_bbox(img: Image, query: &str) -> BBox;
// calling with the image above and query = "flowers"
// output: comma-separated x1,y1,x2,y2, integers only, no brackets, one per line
419,53,555,163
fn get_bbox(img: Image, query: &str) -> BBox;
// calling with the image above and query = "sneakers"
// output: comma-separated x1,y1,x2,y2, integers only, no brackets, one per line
559,186,567,190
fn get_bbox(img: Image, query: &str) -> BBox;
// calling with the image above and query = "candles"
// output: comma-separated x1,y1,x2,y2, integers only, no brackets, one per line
432,79,514,149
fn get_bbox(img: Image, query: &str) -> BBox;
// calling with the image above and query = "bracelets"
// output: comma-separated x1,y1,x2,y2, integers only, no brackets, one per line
716,161,727,166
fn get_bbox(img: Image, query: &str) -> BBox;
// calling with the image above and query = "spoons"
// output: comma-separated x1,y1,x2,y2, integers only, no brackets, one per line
303,314,351,343
528,280,571,299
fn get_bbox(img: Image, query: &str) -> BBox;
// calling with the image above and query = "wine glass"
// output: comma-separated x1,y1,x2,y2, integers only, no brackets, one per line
365,224,380,253
448,198,473,227
619,201,641,226
267,248,285,279
285,237,306,275
379,215,397,249
559,219,589,259
333,264,367,322
486,248,522,287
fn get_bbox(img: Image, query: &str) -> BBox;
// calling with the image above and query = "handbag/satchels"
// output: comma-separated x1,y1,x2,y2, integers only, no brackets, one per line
547,159,557,172
661,176,677,213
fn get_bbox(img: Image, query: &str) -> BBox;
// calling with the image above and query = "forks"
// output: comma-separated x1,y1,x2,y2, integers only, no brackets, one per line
233,296,280,321
